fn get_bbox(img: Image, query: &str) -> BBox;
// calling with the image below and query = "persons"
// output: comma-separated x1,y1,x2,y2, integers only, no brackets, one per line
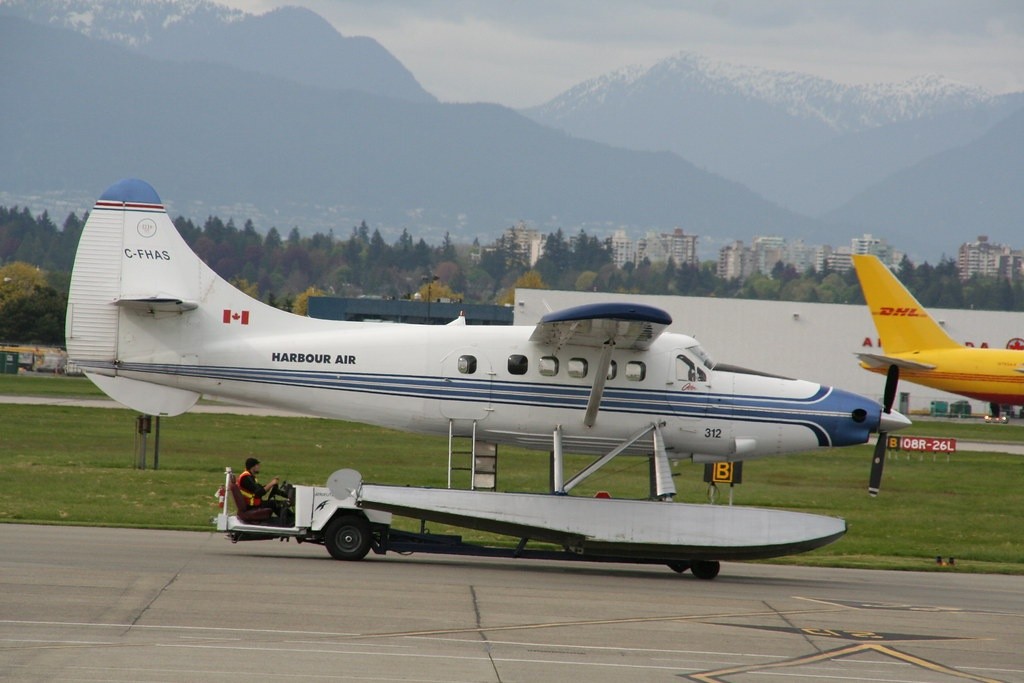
236,457,295,524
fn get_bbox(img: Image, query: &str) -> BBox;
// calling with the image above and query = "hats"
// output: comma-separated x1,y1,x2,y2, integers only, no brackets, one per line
245,458,262,470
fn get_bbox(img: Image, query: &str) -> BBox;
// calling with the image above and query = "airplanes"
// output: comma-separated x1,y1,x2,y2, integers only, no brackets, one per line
64,179,912,503
850,252,1024,425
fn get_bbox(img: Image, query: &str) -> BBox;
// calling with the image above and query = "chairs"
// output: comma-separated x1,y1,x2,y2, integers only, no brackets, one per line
230,475,274,521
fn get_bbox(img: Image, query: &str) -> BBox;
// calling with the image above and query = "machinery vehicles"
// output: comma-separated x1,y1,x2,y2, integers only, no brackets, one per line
214,454,846,579
